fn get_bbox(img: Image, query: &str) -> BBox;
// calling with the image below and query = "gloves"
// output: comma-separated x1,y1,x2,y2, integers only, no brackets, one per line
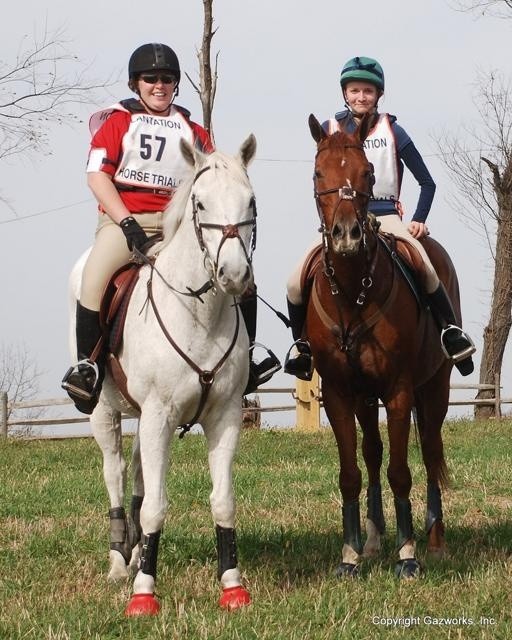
121,218,148,251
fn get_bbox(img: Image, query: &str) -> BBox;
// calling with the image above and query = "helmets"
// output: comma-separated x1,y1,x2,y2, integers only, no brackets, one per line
128,44,179,92
341,57,384,92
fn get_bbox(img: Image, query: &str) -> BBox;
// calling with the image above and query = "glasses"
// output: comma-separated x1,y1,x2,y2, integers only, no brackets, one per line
139,75,175,83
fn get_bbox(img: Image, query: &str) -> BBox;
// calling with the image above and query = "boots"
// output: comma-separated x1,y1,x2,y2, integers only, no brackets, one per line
284,296,315,381
239,281,276,394
426,281,474,376
67,299,106,414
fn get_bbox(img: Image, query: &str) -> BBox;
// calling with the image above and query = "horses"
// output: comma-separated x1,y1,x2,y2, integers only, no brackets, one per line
68,131,258,621
301,111,464,583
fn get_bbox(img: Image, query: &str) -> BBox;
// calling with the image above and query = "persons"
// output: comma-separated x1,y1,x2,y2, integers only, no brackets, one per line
58,41,284,401
284,54,474,382
